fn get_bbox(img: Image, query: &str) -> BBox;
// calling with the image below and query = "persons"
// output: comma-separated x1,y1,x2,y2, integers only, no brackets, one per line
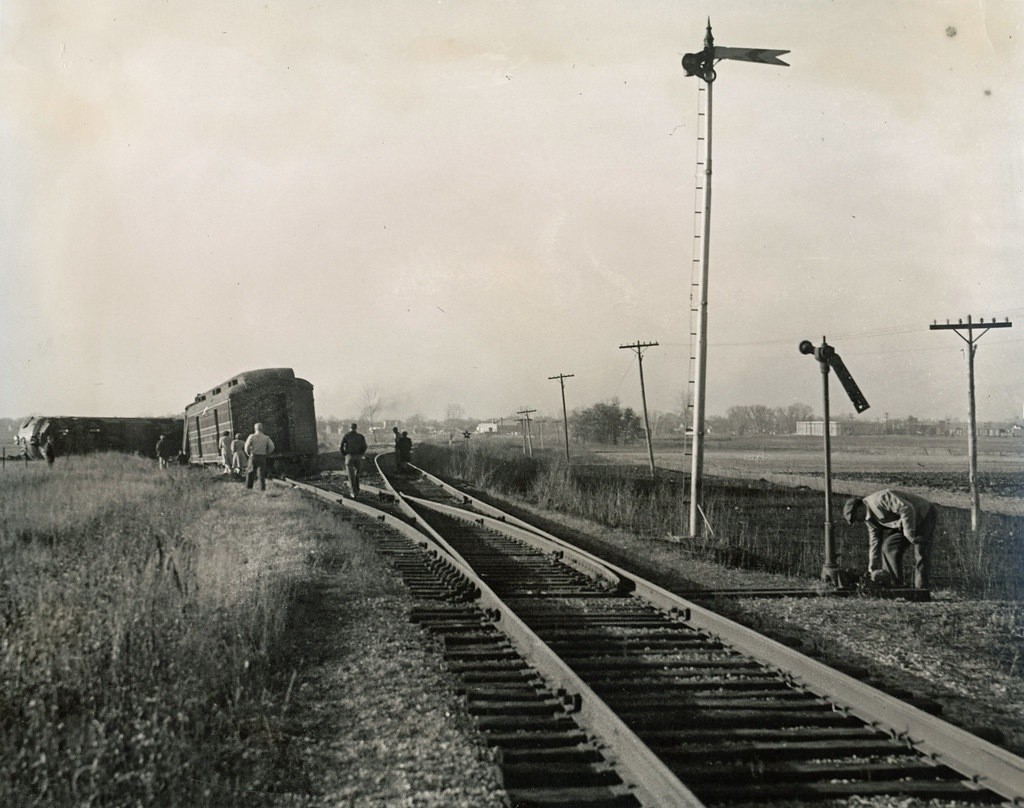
244,423,275,491
156,436,170,470
392,427,412,467
43,436,56,468
231,433,248,474
218,431,233,474
843,489,937,590
339,423,367,498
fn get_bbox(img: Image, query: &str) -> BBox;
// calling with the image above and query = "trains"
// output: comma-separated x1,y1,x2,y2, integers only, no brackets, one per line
181,368,320,472
14,415,185,463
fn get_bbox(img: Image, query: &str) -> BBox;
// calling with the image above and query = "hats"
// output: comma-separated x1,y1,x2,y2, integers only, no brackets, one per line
842,495,864,528
